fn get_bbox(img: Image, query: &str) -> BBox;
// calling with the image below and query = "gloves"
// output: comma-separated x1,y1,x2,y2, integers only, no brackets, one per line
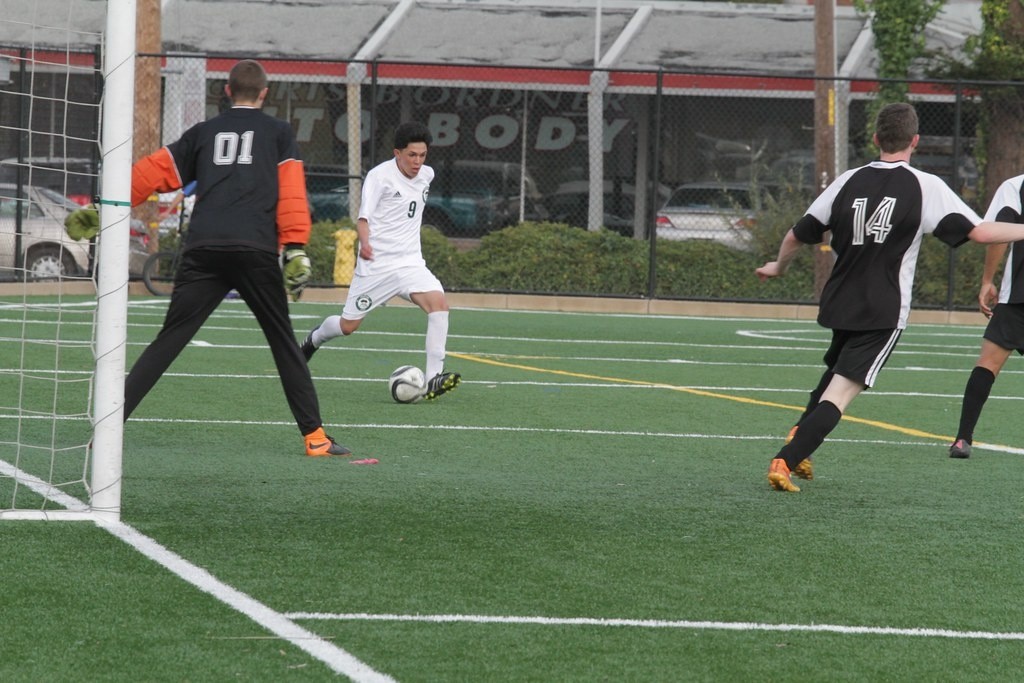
282,242,311,302
64,201,100,241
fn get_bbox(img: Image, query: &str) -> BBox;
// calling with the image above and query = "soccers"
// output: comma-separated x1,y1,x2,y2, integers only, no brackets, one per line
388,365,428,404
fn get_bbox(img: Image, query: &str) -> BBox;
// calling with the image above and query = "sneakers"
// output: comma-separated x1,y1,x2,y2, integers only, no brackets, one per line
768,458,801,492
300,325,320,362
422,368,461,401
786,426,813,479
305,433,353,457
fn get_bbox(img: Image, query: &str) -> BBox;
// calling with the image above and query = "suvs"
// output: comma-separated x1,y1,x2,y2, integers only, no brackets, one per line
308,158,541,233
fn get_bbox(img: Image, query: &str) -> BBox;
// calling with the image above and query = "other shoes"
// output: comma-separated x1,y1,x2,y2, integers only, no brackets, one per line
950,433,972,458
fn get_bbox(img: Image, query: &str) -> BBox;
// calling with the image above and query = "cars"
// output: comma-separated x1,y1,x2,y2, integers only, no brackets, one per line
0,182,152,281
843,142,981,208
0,156,95,205
654,179,784,258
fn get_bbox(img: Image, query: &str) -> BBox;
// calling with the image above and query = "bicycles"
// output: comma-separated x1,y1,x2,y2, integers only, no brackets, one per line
142,198,244,300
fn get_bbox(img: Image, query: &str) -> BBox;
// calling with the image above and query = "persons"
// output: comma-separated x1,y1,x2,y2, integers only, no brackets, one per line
64,58,352,457
755,103,1024,492
949,175,1024,458
299,121,461,402
